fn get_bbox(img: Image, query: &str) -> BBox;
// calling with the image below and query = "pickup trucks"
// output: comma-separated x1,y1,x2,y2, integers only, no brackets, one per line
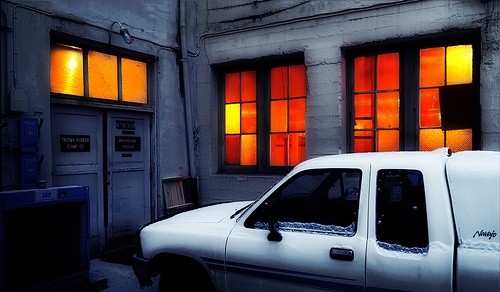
131,146,500,292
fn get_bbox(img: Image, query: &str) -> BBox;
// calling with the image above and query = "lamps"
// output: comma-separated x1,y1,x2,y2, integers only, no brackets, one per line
109,21,132,44
